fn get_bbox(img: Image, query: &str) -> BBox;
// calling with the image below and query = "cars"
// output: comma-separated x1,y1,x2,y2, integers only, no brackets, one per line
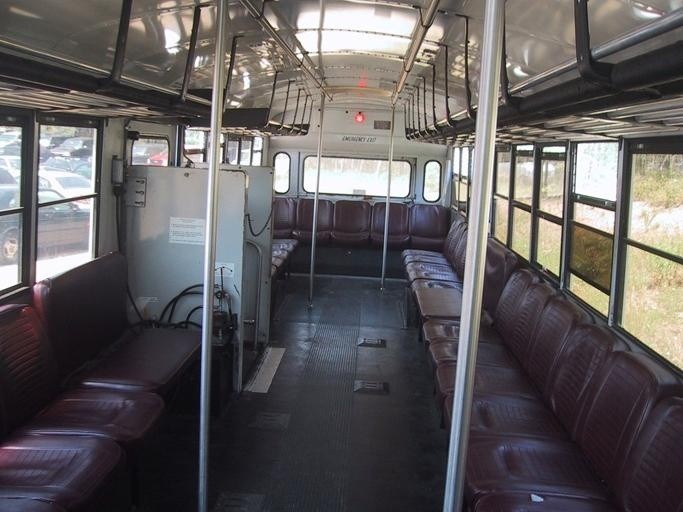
0,129,169,210
0,185,90,264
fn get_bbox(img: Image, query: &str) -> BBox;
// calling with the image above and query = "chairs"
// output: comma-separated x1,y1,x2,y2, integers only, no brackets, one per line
0,300,166,511
272,196,452,253
269,238,297,290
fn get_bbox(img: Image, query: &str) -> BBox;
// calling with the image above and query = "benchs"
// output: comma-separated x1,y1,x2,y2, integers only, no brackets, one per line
32,250,200,398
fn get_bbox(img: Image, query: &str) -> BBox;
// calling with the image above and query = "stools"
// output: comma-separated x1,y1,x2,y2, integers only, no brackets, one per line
0,432,125,511
